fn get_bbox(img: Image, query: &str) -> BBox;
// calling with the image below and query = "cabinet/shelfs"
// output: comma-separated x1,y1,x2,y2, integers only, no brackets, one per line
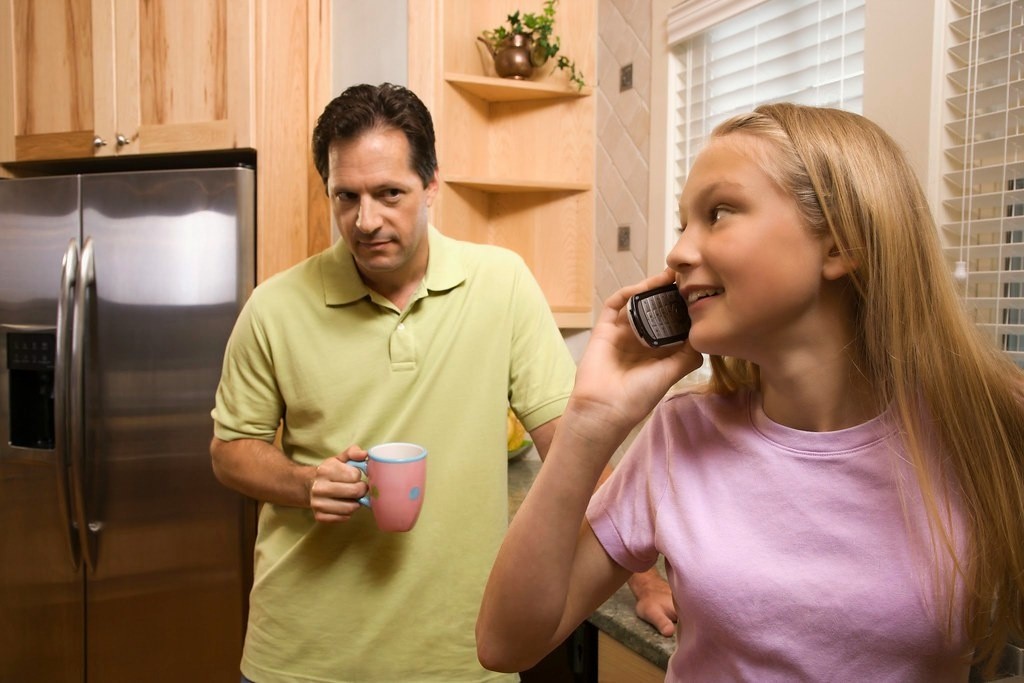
0,0,336,285
438,0,599,407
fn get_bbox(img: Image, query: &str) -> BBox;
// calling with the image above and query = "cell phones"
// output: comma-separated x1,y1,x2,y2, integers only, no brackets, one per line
625,284,691,349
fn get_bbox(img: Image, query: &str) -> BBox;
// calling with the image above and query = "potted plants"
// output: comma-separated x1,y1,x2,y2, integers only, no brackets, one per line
475,1,588,92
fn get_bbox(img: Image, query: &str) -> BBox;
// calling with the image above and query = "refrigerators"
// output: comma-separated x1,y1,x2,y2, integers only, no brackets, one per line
1,168,255,683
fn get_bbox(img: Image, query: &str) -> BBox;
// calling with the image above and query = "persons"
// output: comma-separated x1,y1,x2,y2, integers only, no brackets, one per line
476,103,1024,683
210,83,679,683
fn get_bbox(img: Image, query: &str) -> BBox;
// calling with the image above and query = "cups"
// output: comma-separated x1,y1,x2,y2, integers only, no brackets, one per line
345,442,427,532
